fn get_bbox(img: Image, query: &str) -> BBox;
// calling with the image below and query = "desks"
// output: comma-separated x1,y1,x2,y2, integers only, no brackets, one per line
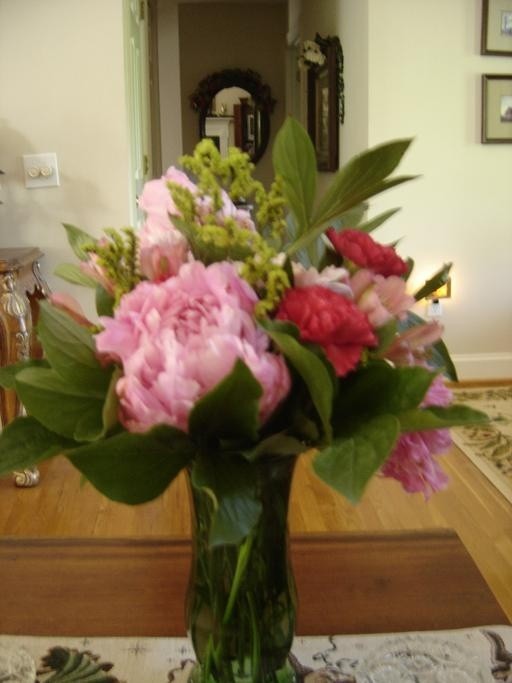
0,246,55,486
0,528,512,683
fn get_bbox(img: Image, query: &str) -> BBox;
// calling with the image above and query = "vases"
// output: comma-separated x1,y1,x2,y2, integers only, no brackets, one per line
180,452,297,683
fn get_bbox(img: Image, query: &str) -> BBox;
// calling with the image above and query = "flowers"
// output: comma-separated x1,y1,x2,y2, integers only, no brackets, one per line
0,118,492,683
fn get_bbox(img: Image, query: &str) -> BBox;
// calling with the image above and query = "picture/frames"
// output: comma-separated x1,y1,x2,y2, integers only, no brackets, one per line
482,74,512,143
298,34,346,172
480,0,512,57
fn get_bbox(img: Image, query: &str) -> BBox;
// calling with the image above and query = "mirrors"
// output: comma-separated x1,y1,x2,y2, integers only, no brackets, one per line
191,67,271,164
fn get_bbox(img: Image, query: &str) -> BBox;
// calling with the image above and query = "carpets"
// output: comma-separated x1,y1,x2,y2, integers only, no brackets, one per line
434,382,512,505
